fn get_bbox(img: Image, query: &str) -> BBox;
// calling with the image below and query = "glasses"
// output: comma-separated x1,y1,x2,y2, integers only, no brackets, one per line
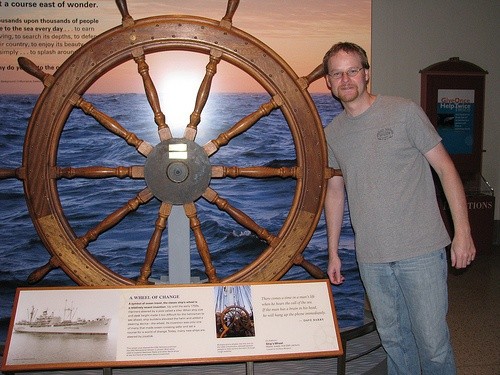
328,67,364,79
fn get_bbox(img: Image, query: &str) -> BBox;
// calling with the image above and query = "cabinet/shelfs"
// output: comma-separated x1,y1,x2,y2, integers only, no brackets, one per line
419,56,495,256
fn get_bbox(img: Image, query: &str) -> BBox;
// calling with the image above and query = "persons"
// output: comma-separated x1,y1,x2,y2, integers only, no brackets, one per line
318,41,477,374
